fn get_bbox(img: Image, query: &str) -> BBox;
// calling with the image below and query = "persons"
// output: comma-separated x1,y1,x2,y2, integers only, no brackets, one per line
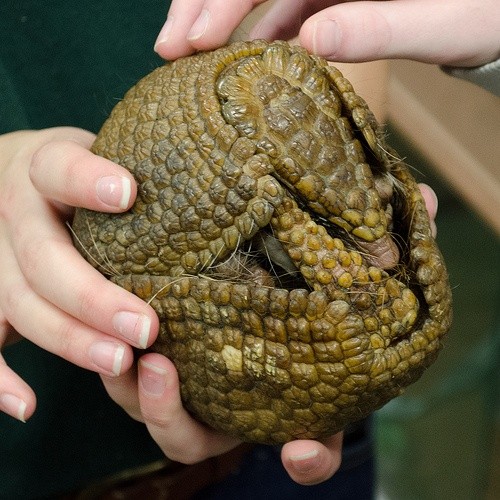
153,0,500,71
0,0,438,500
